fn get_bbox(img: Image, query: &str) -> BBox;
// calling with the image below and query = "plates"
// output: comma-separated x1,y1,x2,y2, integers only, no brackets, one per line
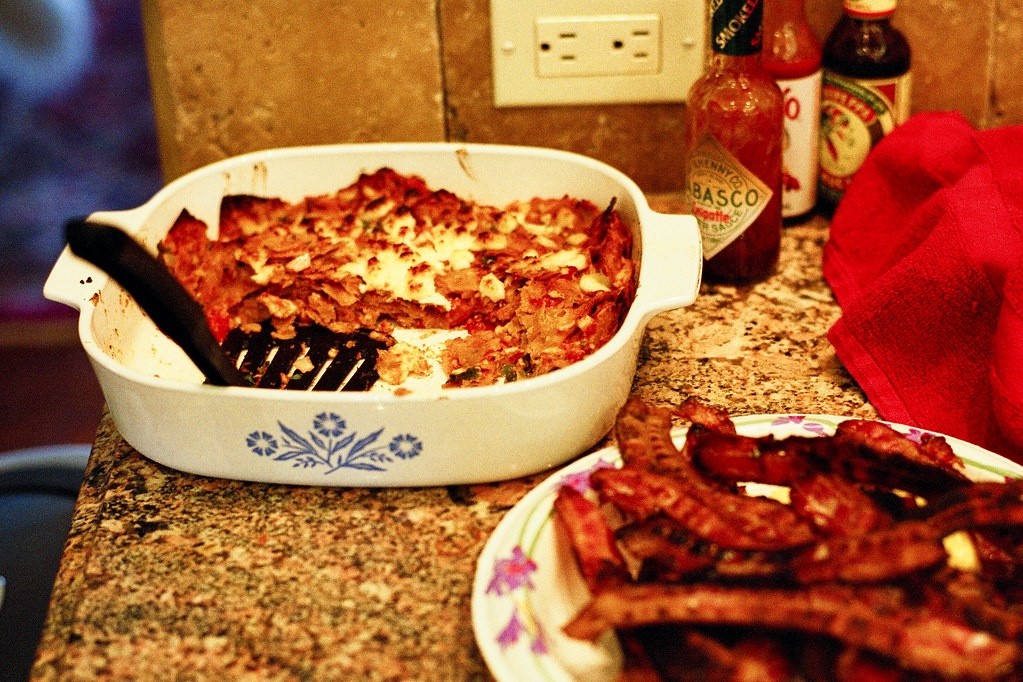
473,412,1023,682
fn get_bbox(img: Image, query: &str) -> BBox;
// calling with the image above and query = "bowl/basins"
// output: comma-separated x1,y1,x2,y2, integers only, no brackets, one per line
41,141,704,488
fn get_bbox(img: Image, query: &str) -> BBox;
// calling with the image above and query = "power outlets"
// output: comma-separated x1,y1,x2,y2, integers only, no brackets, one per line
490,0,710,108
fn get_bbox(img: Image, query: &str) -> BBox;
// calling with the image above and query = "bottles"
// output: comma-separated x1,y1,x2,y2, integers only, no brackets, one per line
762,3,823,230
814,0,915,225
685,0,782,285
